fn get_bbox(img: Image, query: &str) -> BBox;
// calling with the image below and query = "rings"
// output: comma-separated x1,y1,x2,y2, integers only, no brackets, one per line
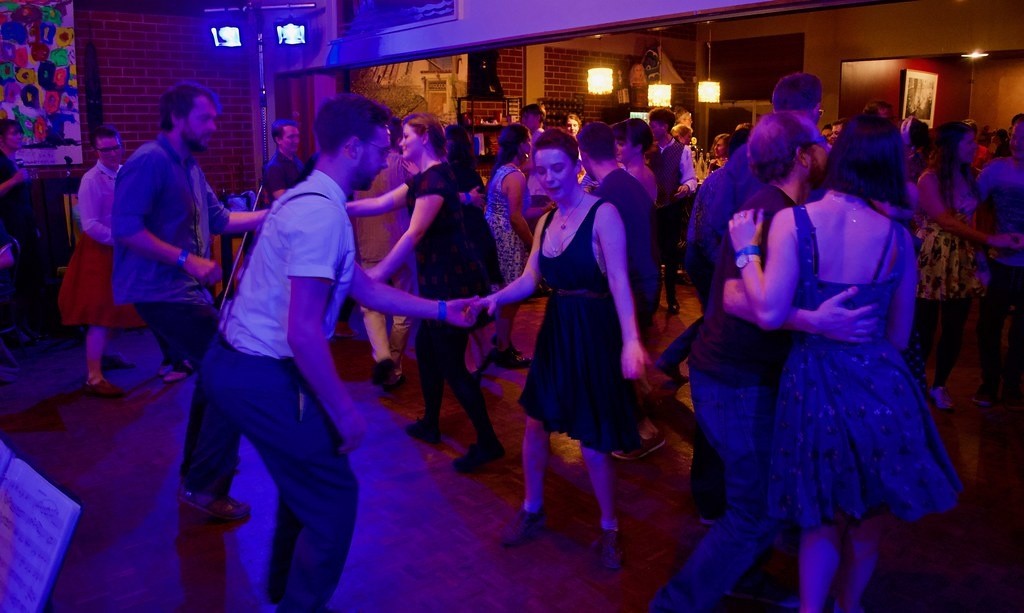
740,215,745,218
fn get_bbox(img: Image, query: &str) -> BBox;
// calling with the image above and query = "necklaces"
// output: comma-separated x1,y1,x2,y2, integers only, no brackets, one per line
561,191,585,230
832,192,864,223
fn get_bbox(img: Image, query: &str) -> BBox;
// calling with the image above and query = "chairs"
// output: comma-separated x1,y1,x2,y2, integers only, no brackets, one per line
0,236,31,369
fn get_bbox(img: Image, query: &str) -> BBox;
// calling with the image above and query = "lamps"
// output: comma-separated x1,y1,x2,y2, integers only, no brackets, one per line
587,34,613,95
697,22,720,103
647,29,671,108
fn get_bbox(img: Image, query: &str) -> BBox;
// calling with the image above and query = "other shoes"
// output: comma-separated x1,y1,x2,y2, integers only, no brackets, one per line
383,377,406,394
488,345,532,369
177,486,250,518
930,386,956,410
160,356,176,375
164,360,194,383
600,521,625,569
667,301,682,316
99,351,138,369
84,378,123,398
971,394,998,407
611,430,664,460
655,355,690,382
451,438,507,471
490,334,524,356
500,506,546,547
371,357,395,385
404,418,441,445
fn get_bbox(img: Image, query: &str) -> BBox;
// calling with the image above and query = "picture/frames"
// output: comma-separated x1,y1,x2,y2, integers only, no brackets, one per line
900,69,938,129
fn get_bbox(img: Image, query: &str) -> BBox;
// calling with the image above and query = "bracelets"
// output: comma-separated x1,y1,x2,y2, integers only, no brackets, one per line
463,191,472,205
734,245,760,259
437,300,448,320
177,249,190,267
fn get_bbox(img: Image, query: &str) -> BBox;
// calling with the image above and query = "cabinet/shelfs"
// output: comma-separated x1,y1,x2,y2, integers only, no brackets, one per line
458,94,522,163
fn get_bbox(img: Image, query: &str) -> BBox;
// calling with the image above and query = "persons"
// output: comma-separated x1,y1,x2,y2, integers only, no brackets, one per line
0,73,1024,613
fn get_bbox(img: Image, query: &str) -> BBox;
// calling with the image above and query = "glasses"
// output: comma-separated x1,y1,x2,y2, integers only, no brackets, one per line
345,139,391,159
789,136,827,162
93,143,120,152
817,109,825,117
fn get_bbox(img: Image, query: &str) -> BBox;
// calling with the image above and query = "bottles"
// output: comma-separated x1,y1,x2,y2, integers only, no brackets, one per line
689,142,711,183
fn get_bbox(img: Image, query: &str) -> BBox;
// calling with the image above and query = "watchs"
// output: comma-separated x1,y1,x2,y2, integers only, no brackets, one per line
736,254,761,270
